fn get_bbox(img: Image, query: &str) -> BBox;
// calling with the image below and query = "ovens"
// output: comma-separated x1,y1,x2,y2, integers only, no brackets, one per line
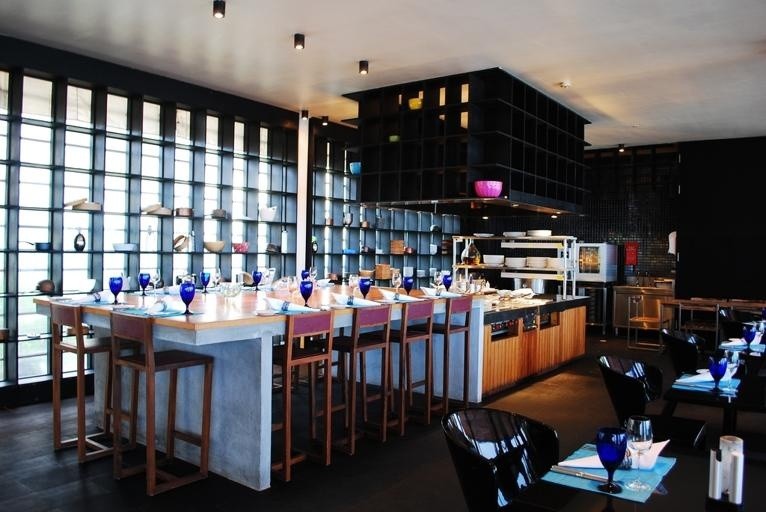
577,287,607,336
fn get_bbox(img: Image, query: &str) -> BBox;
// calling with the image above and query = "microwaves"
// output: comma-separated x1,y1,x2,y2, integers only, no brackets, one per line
573,242,618,284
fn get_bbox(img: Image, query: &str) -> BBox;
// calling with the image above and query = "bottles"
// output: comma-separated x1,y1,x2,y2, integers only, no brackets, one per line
281,230,288,252
461,239,481,265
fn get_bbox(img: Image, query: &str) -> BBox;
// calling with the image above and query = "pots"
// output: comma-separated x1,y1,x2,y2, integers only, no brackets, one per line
18,240,51,251
522,279,548,295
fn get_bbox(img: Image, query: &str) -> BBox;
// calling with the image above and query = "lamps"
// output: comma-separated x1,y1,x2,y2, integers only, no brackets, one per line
359,61,367,75
302,110,308,119
214,0,225,19
322,116,329,125
618,143,624,152
294,33,304,50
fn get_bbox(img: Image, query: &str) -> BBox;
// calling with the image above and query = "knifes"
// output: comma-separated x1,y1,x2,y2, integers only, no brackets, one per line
551,465,607,483
674,383,713,388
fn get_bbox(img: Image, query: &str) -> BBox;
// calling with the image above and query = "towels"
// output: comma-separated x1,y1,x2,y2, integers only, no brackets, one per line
147,293,174,315
72,290,115,305
265,286,464,312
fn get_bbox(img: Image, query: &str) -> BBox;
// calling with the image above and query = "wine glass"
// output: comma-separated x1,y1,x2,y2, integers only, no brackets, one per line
109,277,123,304
349,274,359,295
150,269,161,296
596,426,622,494
724,348,739,393
403,277,413,295
138,273,150,296
708,356,727,393
392,273,402,300
626,416,652,491
301,270,309,280
443,275,452,291
434,272,443,295
252,272,262,291
742,323,756,352
309,267,317,281
359,279,371,299
287,276,299,302
179,284,195,316
300,282,313,308
200,272,210,293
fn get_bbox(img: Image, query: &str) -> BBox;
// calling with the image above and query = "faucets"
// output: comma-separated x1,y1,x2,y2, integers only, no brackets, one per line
635,269,640,287
645,270,652,287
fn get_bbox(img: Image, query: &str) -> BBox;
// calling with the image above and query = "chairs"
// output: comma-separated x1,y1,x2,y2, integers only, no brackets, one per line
50,303,143,463
272,311,335,480
110,311,215,496
359,299,434,436
309,304,392,453
407,297,473,415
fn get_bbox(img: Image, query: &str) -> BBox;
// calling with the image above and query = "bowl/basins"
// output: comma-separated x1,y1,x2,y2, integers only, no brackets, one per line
360,270,374,277
113,243,136,251
203,241,226,252
429,268,437,276
474,180,503,197
349,162,361,174
430,245,437,255
442,270,451,275
218,281,244,297
404,266,413,277
233,242,249,253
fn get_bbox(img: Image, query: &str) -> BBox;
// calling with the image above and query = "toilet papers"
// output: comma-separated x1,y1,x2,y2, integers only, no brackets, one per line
719,435,744,495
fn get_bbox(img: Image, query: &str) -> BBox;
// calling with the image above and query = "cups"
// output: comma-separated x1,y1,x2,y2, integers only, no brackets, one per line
460,283,467,292
318,286,332,306
469,284,475,293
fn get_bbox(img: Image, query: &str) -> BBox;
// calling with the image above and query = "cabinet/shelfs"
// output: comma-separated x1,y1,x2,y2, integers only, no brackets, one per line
341,67,591,218
612,277,676,336
306,117,465,279
451,236,577,297
0,37,298,409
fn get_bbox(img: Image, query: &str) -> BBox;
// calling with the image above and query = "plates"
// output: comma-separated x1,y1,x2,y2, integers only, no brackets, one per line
503,232,526,238
505,258,527,267
474,234,494,237
483,254,504,264
527,257,550,267
417,270,426,276
256,310,276,316
527,230,552,237
547,258,567,269
